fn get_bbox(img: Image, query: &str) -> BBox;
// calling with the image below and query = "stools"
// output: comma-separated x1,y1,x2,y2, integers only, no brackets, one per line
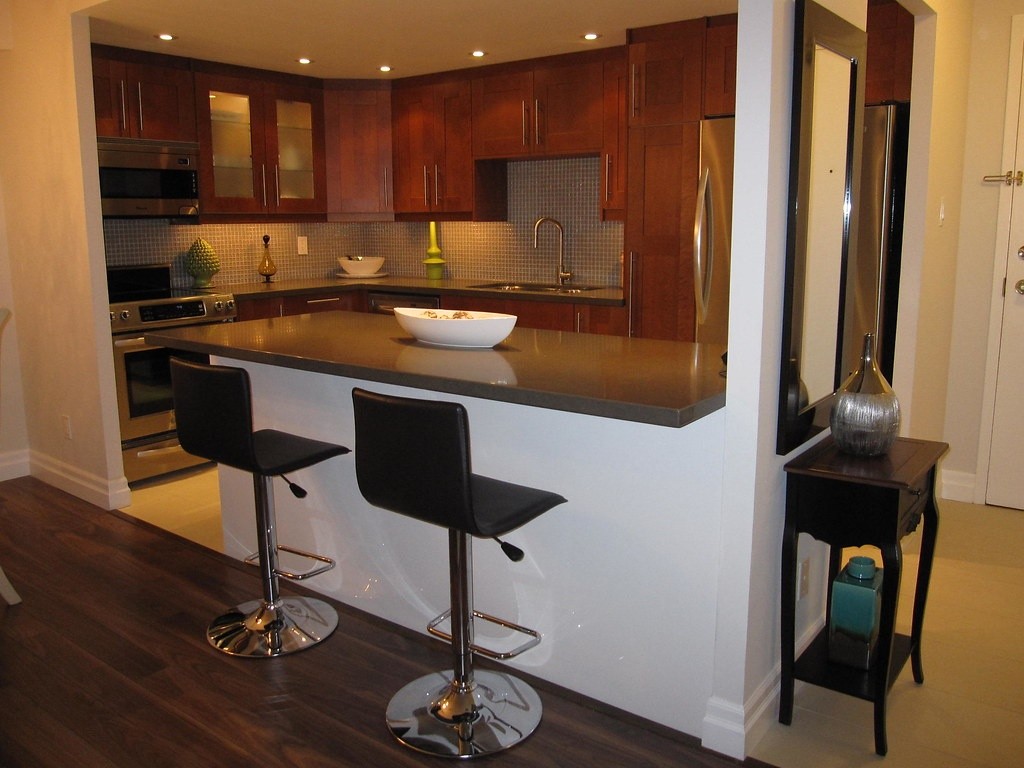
168,355,350,660
348,388,566,761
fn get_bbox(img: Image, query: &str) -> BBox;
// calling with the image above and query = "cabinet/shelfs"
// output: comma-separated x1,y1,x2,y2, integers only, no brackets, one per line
780,432,948,755
90,12,738,340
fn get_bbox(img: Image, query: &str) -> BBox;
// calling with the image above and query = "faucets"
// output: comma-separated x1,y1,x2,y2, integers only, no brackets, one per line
534,217,573,284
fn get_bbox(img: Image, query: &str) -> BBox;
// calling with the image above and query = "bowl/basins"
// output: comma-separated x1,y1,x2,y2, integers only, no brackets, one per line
338,256,385,274
393,306,518,351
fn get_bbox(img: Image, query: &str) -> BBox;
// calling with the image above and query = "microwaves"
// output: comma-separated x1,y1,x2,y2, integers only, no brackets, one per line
95,136,200,218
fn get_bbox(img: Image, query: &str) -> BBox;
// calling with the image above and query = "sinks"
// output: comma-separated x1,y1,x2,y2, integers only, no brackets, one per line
465,282,542,290
526,284,604,293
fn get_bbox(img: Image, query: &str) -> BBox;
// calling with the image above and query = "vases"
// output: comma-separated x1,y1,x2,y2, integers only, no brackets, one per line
827,332,902,456
829,552,885,669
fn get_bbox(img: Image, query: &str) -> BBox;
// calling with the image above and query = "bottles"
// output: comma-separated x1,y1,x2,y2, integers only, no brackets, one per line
829,333,902,458
828,556,883,671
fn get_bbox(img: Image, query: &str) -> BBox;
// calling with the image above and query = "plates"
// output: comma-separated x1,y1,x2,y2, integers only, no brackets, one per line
336,273,389,278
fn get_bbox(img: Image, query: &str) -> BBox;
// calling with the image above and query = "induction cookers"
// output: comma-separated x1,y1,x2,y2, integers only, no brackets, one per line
106,262,238,333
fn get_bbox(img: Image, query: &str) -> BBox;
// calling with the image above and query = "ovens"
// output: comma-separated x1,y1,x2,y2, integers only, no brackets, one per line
111,333,210,482
